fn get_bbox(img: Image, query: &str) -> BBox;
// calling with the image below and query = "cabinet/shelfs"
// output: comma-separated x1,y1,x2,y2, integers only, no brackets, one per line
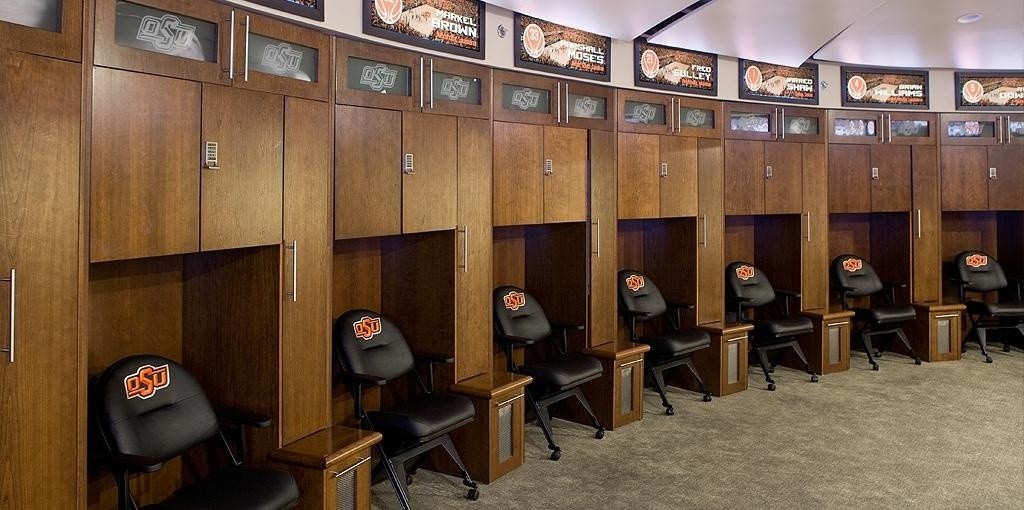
333,35,493,117
618,89,725,138
723,139,802,215
697,321,755,398
580,339,652,430
940,145,1023,210
383,114,493,384
446,370,533,485
616,131,698,219
333,105,456,239
939,113,1024,145
645,136,724,325
267,424,383,509
0,47,84,508
492,120,587,226
0,1,84,63
909,298,967,363
756,139,830,311
801,308,857,375
723,101,826,141
92,1,330,103
492,68,616,131
826,109,937,144
186,94,335,448
827,144,911,211
872,143,942,303
89,64,284,260
526,129,618,347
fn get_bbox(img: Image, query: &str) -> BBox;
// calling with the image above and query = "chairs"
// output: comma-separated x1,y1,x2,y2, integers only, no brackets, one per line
87,351,304,508
616,269,714,417
724,261,819,391
831,253,922,370
493,286,606,461
952,250,1024,363
331,311,480,510
